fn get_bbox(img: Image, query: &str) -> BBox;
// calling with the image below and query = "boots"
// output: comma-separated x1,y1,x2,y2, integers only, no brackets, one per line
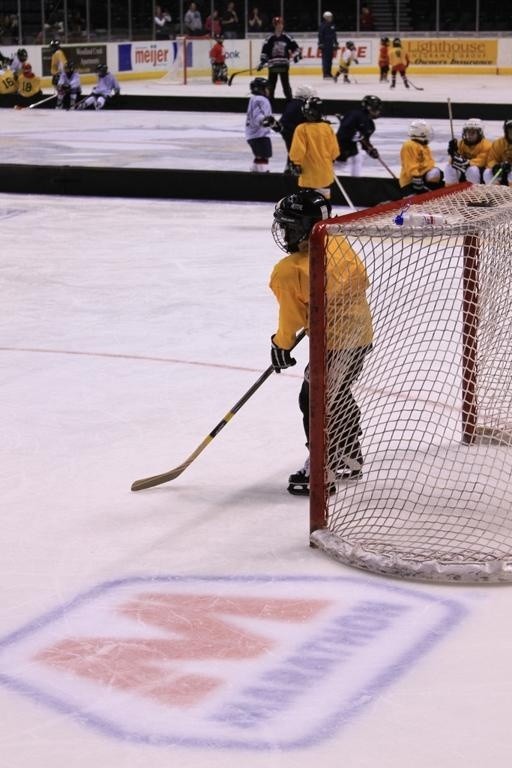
327,430,366,473
76,101,101,111
332,70,410,88
287,441,336,487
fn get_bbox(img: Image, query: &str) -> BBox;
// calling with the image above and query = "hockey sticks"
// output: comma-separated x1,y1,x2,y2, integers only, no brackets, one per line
65,95,90,108
407,78,424,91
130,326,308,494
228,68,259,87
14,93,58,110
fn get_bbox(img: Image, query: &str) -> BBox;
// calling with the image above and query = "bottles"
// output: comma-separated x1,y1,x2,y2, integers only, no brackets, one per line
393,211,444,226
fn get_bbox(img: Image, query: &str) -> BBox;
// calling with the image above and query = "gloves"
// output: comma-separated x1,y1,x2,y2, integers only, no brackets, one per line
410,138,511,191
269,333,297,374
358,138,380,160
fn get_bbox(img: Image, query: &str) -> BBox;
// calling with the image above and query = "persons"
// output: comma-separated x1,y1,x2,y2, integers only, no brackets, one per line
155,3,410,114
0,0,119,110
269,190,373,485
244,78,512,202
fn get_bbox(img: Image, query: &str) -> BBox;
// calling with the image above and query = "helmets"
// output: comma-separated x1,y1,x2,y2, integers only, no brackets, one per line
406,118,512,144
322,10,334,19
0,39,109,76
300,95,324,122
272,186,334,247
345,40,354,48
380,36,401,48
359,94,384,113
271,16,285,25
249,76,274,95
294,84,317,102
214,31,225,41
